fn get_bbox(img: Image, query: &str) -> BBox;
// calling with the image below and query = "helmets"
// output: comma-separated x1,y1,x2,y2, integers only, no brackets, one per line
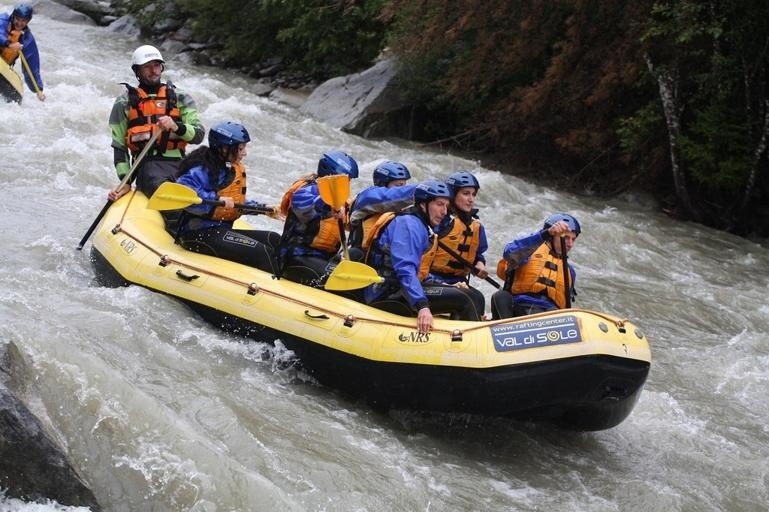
543,214,580,237
318,151,358,178
446,171,479,192
209,122,250,147
374,160,410,185
131,44,165,73
415,179,455,200
14,4,32,19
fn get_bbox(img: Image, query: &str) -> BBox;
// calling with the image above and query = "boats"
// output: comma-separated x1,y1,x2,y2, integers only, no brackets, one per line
90,183,652,433
0,51,28,104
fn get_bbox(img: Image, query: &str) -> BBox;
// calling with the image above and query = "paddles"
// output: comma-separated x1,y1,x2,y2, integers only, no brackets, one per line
317,174,350,261
324,260,457,291
145,182,277,217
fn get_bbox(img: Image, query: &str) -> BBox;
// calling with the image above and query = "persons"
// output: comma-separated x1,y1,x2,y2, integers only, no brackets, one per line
278,150,365,288
162,121,283,277
105,44,205,201
0,3,45,101
491,213,581,320
430,171,488,315
347,160,419,262
360,179,480,331
